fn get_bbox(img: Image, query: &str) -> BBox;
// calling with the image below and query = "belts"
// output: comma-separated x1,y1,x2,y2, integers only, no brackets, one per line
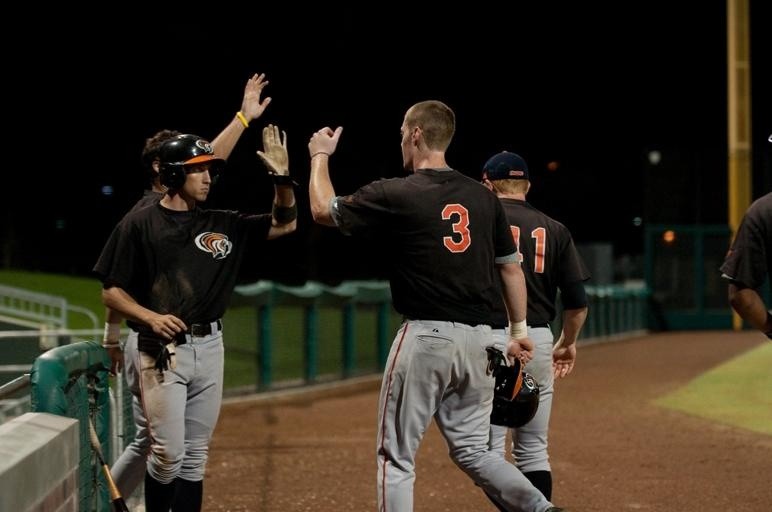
183,320,222,337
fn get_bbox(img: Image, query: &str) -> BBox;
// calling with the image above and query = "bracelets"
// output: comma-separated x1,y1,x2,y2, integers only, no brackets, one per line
506,320,528,338
269,176,296,185
272,207,298,226
234,109,249,129
307,151,330,159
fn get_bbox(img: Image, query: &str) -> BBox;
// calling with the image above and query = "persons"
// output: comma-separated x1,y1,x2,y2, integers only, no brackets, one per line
479,149,591,501
106,122,298,511
306,99,565,512
719,193,771,339
111,69,272,505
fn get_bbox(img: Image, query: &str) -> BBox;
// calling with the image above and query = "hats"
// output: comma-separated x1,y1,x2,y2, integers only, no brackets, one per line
479,150,529,181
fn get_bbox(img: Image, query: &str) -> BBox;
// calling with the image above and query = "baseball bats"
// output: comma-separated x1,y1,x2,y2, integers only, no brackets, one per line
89,417,130,512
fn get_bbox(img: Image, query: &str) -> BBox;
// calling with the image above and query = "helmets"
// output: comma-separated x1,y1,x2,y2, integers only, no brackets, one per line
489,356,540,429
158,133,227,190
